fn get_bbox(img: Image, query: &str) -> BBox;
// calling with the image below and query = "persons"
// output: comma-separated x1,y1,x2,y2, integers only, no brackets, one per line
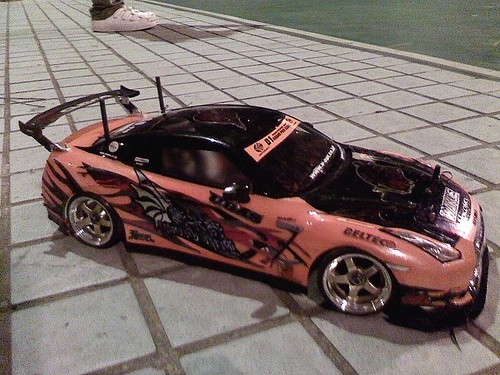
89,0,157,33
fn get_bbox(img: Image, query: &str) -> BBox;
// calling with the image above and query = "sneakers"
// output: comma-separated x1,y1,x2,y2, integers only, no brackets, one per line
123,5,156,19
91,7,158,31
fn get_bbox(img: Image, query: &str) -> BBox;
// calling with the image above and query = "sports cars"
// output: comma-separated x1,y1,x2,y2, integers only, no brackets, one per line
18,76,488,319
